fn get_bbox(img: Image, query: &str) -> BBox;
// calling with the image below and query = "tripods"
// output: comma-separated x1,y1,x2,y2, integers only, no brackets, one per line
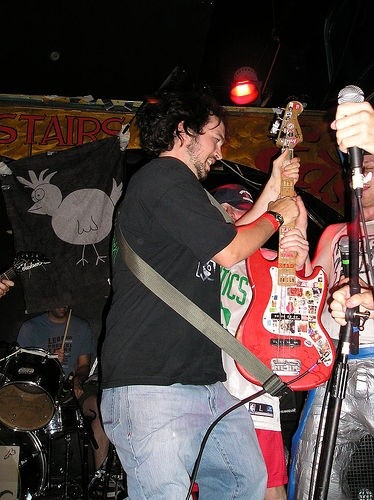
54,433,86,500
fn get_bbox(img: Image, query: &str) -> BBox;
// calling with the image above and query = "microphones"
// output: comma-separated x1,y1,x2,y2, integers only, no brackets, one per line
336,85,365,198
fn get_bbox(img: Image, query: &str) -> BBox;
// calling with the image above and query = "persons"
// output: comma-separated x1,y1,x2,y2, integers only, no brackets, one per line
0,191,27,345
17,306,109,468
191,185,309,500
295,102,374,500
99,94,300,500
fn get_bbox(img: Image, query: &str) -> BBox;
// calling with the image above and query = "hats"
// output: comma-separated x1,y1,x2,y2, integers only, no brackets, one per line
214,184,254,211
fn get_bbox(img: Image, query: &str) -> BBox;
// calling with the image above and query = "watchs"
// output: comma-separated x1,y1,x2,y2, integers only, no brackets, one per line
268,211,285,227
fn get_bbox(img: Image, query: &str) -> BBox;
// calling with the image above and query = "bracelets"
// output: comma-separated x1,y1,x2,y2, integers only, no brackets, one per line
263,214,278,232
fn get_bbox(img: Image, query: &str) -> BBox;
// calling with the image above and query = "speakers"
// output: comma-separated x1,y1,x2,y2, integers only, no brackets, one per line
0,444,23,500
347,434,374,499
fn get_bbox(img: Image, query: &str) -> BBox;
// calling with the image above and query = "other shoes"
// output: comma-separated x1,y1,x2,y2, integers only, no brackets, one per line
92,483,121,497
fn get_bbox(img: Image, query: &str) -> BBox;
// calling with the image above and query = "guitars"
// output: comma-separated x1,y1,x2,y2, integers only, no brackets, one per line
234,99,336,391
0,251,54,284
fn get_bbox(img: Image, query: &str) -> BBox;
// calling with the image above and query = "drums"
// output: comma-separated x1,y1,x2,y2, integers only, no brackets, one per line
0,347,65,431
42,405,84,432
0,426,48,500
